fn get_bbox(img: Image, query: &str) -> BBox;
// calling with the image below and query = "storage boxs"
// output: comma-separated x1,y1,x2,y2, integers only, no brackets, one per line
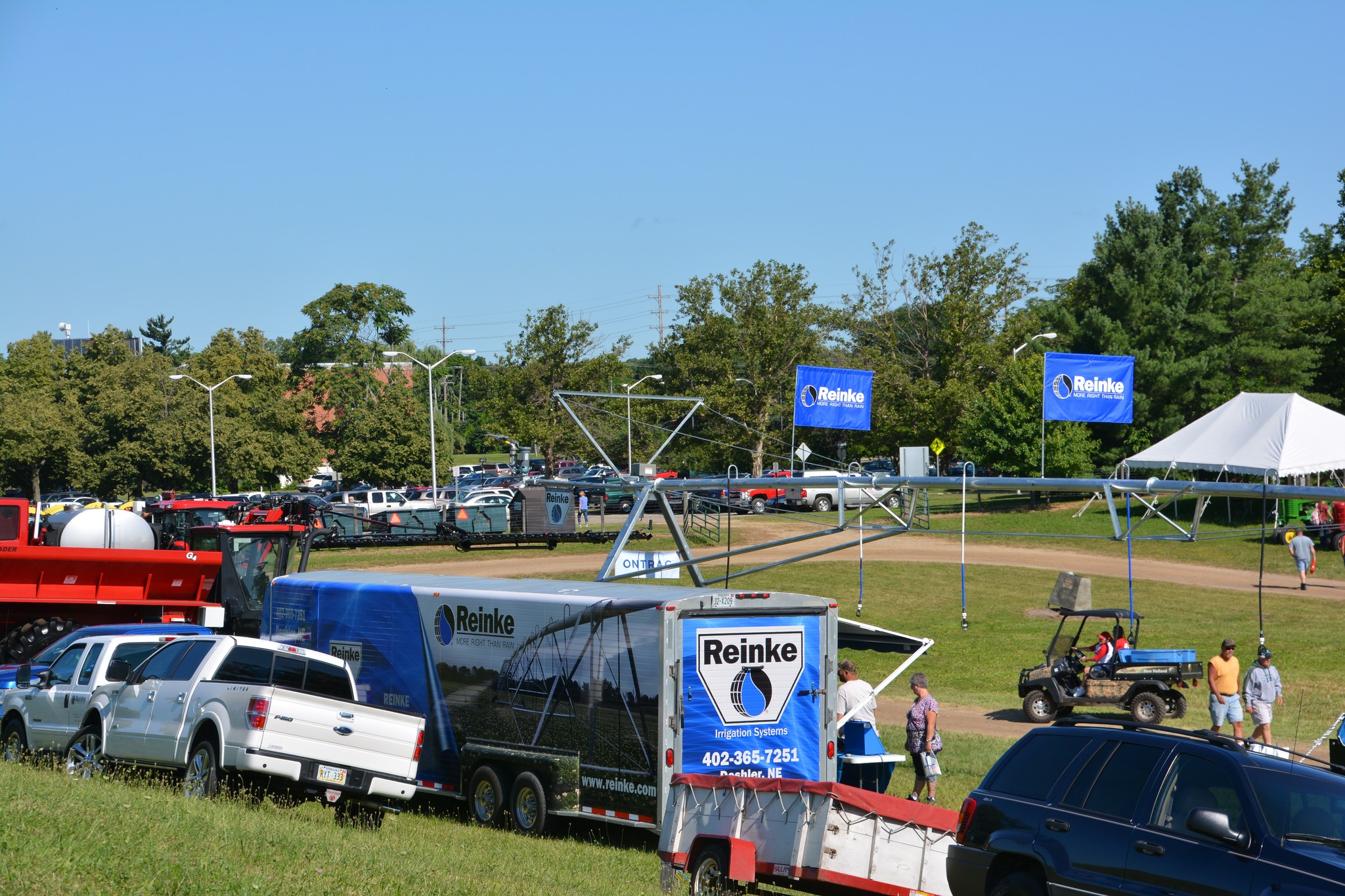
1131,649,1197,663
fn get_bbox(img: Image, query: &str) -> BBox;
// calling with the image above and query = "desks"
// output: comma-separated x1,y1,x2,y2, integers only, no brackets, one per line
838,748,907,793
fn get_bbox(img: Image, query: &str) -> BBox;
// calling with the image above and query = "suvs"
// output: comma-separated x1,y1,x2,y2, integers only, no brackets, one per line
404,455,903,521
296,474,407,497
0,622,214,766
175,492,367,519
944,718,1345,896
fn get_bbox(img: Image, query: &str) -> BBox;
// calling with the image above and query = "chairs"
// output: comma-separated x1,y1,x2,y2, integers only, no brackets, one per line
1242,785,1291,840
1089,652,1118,679
1168,760,1218,837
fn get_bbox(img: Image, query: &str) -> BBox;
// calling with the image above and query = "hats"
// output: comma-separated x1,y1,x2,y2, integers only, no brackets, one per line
837,660,857,672
1258,647,1273,658
1221,639,1237,647
1097,631,1111,640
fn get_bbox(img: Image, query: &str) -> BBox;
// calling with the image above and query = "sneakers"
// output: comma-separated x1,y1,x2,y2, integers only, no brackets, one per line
1069,687,1080,694
585,525,588,527
577,525,581,528
1073,686,1085,697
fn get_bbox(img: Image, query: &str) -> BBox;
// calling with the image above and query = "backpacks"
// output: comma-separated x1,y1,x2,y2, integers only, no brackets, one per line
1310,503,1323,525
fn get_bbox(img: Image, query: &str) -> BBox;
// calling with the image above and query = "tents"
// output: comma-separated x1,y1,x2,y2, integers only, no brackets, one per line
1072,392,1345,527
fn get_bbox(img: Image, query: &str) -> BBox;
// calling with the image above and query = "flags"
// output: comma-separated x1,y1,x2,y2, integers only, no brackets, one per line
795,365,873,430
1044,353,1135,423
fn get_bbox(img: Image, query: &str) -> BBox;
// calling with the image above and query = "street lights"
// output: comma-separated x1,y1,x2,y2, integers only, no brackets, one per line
1013,332,1057,494
169,375,253,496
381,350,478,501
621,375,662,474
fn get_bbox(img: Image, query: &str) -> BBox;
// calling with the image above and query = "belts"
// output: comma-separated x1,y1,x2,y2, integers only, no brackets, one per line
1211,691,1233,697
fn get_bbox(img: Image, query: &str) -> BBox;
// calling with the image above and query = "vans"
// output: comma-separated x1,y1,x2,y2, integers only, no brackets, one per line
31,485,126,512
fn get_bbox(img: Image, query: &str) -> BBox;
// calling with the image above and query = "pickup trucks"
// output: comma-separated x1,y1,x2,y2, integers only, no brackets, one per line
1018,606,1203,725
62,635,425,833
309,490,436,521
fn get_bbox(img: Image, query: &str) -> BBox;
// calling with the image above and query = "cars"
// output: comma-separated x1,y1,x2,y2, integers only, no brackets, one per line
930,459,993,477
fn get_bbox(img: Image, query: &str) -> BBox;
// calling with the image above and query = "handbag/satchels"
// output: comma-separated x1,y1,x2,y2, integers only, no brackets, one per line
1310,558,1316,574
923,732,936,741
920,749,942,778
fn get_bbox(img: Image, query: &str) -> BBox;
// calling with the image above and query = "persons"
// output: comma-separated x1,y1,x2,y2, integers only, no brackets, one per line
153,494,162,503
346,496,355,506
1341,535,1345,568
1288,529,1316,590
1069,631,1114,697
904,673,943,806
837,659,877,742
1207,639,1244,746
578,491,588,528
1242,648,1283,745
1318,501,1334,550
1112,626,1129,651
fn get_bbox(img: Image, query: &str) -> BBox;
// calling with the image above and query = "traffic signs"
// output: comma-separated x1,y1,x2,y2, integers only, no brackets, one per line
836,442,847,447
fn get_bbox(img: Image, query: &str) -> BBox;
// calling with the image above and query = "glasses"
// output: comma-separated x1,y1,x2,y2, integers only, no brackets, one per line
1113,631,1117,633
1224,645,1234,650
911,683,918,688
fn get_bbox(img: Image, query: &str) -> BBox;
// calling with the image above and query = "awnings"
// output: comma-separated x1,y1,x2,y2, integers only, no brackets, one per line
838,617,935,728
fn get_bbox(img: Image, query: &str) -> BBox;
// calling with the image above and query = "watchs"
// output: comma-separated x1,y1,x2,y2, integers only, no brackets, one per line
926,739,931,742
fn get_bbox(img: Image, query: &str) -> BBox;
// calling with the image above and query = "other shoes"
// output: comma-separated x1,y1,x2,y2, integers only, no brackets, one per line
926,796,936,801
1300,583,1307,590
905,794,918,802
